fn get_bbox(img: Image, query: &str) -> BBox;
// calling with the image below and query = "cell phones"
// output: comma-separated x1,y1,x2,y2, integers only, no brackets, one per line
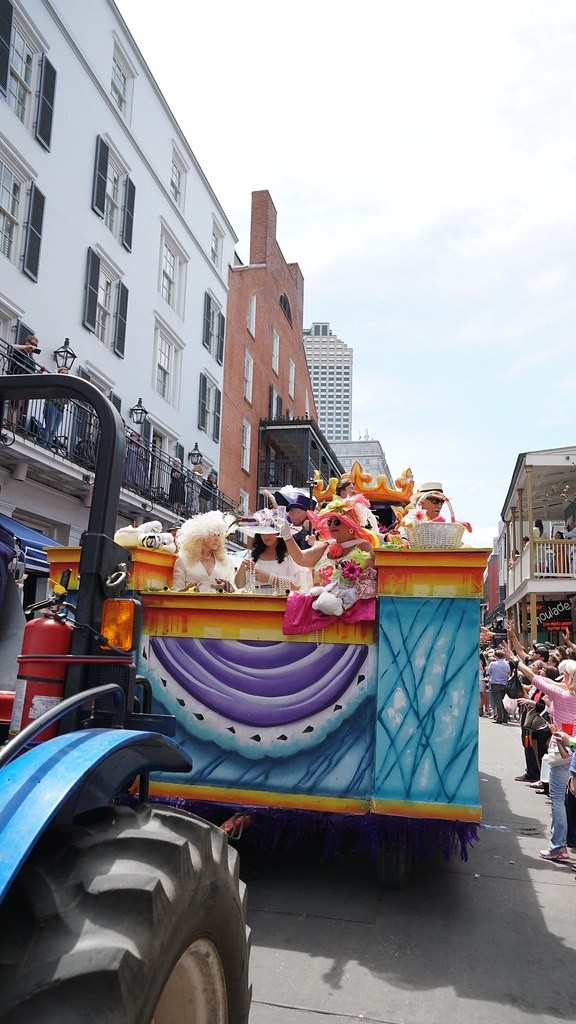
32,348,42,354
540,710,551,724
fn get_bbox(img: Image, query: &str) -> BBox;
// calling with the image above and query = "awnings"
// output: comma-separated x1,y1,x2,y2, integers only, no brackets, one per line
0,513,65,572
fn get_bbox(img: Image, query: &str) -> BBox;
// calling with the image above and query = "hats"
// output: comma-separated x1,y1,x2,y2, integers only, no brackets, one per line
237,514,303,537
532,644,550,660
286,494,317,513
408,482,451,505
555,659,575,681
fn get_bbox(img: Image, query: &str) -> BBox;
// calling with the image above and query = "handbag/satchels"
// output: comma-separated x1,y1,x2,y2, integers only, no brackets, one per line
524,706,547,733
502,694,518,714
547,744,570,768
504,664,524,699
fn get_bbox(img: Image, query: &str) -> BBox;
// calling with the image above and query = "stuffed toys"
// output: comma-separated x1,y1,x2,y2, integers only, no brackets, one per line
310,583,343,616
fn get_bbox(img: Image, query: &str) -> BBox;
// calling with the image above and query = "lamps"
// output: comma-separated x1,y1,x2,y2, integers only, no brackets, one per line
188,443,203,465
129,398,148,423
52,338,77,370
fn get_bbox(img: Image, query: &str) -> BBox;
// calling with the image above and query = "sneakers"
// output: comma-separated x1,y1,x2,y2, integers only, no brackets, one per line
537,846,569,860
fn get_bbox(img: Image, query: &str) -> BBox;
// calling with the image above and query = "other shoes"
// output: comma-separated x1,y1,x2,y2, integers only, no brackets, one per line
479,707,511,724
514,774,541,783
537,787,549,794
529,780,546,787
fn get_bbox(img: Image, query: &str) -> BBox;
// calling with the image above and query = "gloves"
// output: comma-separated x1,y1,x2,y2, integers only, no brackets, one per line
342,587,360,610
274,518,292,541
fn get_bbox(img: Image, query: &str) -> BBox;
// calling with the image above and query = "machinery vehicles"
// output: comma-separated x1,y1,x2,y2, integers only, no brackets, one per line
0,372,255,1023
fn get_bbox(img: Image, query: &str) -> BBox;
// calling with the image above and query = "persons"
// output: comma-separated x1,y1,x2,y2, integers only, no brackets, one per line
43,364,69,447
6,335,39,430
187,463,204,513
276,511,375,589
123,430,148,494
235,508,302,595
172,510,236,593
507,519,576,578
170,457,185,515
259,478,444,550
479,621,576,874
199,474,218,512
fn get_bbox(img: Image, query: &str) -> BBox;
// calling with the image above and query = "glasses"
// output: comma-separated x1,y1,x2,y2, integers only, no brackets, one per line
27,340,37,347
325,519,345,526
534,650,540,655
426,497,445,506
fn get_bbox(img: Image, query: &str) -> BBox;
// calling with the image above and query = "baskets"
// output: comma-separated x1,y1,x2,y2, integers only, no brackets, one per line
406,491,465,549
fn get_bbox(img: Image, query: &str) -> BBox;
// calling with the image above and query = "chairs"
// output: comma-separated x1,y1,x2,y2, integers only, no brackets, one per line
21,413,173,507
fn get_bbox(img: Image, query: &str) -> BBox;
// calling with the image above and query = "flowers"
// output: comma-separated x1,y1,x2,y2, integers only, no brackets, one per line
313,543,371,586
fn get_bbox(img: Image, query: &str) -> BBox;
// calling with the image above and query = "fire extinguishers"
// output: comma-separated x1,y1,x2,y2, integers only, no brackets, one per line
8,568,75,750
